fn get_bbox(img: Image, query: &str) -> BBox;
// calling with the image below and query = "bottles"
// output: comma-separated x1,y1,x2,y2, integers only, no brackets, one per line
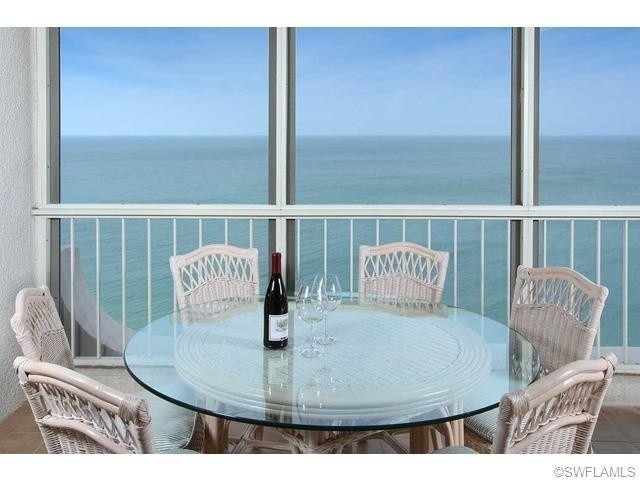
263,252,289,350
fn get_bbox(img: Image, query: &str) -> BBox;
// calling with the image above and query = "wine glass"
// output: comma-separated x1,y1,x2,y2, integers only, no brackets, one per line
297,277,327,358
313,274,342,346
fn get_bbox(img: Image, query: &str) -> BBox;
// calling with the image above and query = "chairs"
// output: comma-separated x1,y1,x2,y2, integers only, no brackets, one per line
10,243,618,454
